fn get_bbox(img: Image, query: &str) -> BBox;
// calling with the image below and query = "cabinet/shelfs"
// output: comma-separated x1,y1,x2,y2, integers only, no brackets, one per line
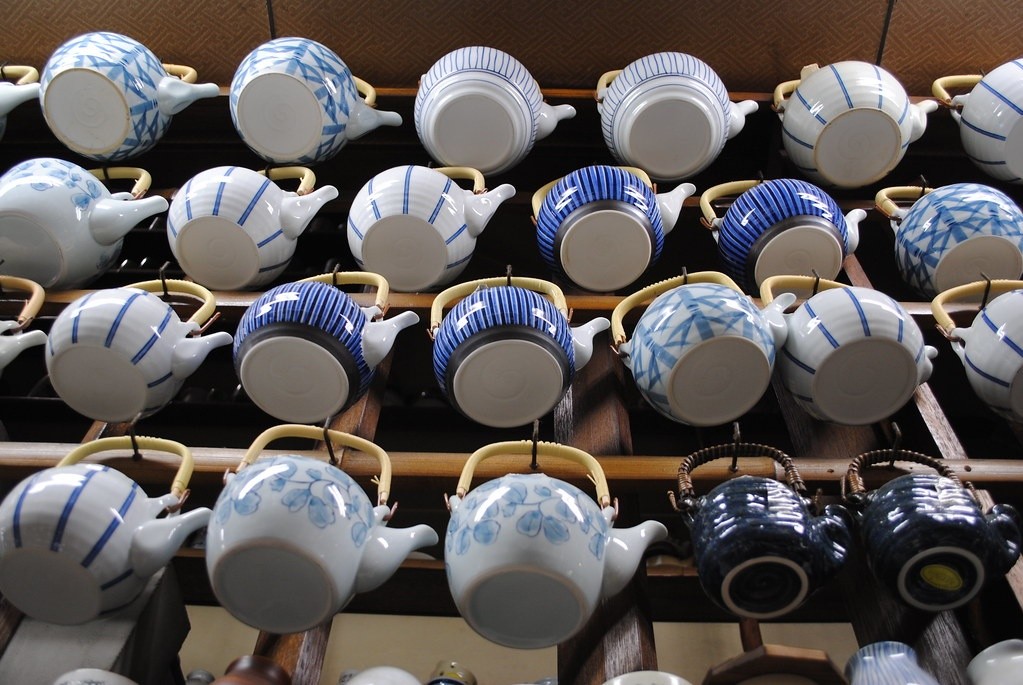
0,64,1023,685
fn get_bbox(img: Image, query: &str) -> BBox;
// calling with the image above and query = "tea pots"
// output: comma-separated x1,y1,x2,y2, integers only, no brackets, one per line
1,31,1021,685
